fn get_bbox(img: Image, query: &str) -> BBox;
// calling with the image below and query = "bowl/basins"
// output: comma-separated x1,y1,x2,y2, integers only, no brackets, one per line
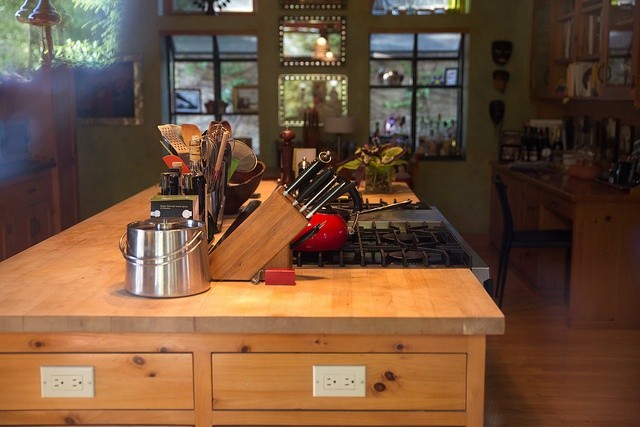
224,160,266,214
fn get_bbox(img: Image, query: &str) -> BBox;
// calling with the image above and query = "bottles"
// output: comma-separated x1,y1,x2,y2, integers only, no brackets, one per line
162,160,193,194
519,125,562,162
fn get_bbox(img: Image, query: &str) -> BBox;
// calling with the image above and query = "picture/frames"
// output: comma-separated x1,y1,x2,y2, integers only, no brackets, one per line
71,53,144,127
278,74,351,127
279,1,349,10
278,15,348,67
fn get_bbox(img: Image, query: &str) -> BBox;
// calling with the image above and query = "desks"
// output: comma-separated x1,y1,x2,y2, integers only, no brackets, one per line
490,158,640,330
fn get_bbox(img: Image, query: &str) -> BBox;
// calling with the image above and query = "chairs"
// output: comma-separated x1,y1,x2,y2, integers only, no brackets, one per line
483,152,572,309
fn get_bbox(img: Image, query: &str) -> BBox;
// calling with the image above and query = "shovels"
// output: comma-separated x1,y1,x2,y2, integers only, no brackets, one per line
158,124,190,171
181,123,200,149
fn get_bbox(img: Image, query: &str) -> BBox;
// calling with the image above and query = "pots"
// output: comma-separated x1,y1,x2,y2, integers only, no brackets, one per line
293,200,412,254
116,217,212,299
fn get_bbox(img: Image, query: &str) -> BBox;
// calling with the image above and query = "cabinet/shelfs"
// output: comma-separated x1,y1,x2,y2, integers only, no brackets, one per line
36,65,83,230
1,177,53,262
531,1,635,103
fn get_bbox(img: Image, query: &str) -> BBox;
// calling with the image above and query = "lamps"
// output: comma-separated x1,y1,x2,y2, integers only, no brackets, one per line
323,117,357,162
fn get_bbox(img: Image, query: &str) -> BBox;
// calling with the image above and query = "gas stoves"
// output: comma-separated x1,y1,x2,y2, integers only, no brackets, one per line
292,197,492,287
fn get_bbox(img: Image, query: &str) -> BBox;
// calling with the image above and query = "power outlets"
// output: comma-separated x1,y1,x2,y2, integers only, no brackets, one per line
41,365,95,399
313,365,366,397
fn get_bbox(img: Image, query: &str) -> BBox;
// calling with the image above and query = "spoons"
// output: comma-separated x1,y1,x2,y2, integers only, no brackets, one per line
182,120,231,189
